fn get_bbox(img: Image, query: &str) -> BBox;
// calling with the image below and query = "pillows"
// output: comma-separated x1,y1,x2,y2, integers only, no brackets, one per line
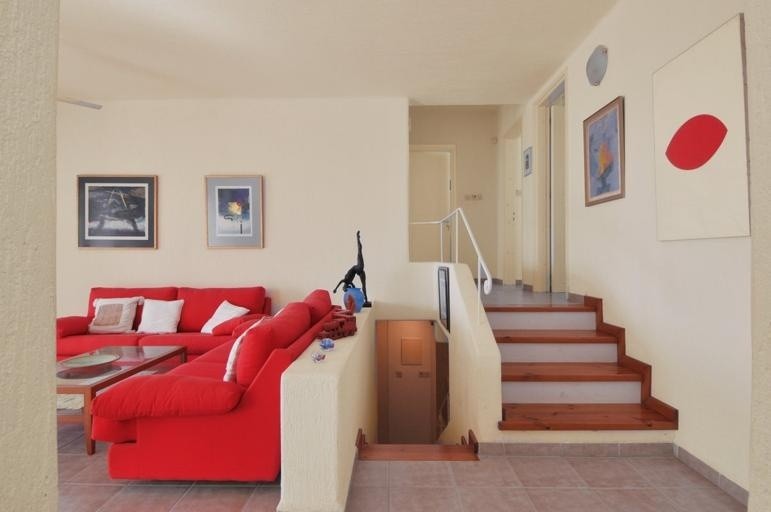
137,299,184,335
224,317,266,384
89,295,144,334
199,301,250,334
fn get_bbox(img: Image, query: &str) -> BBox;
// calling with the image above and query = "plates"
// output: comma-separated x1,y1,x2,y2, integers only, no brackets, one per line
57,363,118,379
58,352,121,369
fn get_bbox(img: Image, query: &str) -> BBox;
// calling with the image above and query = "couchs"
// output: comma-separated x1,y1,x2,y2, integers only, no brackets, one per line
54,288,272,370
91,290,342,481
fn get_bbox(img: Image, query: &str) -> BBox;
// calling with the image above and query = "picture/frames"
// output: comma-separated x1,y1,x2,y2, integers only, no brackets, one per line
522,146,534,178
438,266,450,332
76,174,158,250
204,175,263,251
584,96,625,206
